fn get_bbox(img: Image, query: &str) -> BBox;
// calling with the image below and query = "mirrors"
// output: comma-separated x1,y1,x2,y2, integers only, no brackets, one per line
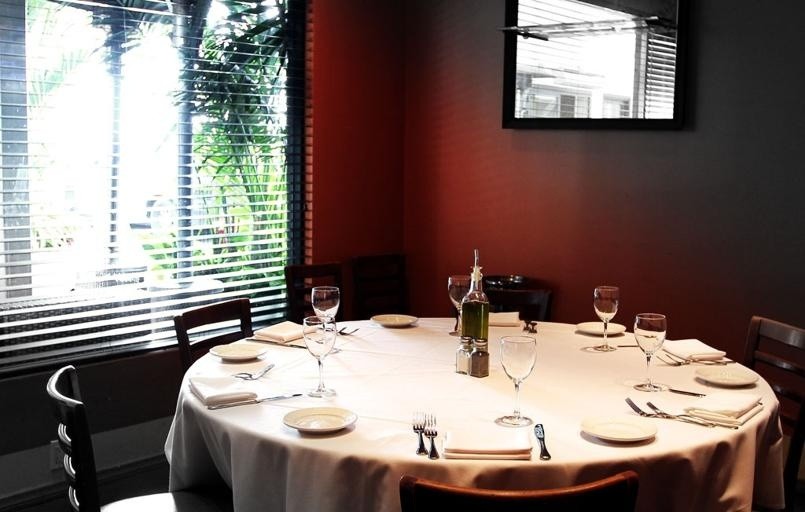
502,0,692,130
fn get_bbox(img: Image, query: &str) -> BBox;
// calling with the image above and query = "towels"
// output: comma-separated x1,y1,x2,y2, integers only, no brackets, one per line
664,334,727,365
443,427,537,460
486,310,521,327
249,319,316,344
188,372,259,408
684,389,765,424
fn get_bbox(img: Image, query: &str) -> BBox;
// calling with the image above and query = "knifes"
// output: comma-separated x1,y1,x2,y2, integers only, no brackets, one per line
534,423,552,461
207,392,301,411
669,387,707,397
246,337,307,351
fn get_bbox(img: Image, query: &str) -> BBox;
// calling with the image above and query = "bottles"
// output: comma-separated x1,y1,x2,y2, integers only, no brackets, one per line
461,266,490,345
454,335,491,378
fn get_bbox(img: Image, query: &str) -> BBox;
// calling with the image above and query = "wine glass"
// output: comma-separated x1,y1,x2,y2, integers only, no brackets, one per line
446,274,471,338
590,285,620,354
499,335,537,427
303,313,339,401
631,312,669,392
311,285,342,354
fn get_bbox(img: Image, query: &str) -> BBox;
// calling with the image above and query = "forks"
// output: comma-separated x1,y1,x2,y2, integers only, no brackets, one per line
410,409,440,462
626,396,739,430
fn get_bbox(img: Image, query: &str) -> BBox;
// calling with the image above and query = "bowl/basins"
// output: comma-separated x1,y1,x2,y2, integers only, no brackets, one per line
483,273,547,290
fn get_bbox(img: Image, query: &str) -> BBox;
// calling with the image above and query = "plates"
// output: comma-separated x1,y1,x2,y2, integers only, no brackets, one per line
210,343,270,361
578,320,626,336
694,363,761,389
579,417,657,445
371,314,418,328
282,408,358,435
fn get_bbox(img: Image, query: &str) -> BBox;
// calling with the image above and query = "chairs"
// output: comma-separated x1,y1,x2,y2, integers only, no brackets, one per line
46,363,217,511
399,469,642,512
481,273,558,321
171,250,408,371
745,315,804,512
